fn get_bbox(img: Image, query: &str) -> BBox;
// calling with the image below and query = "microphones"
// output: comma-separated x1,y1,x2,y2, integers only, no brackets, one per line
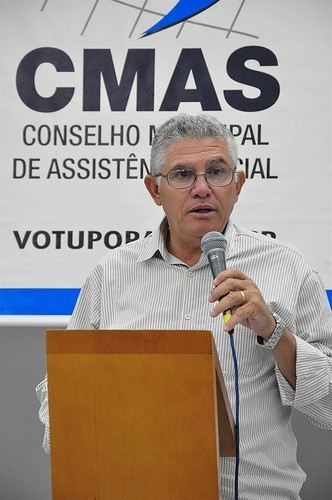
201,232,236,334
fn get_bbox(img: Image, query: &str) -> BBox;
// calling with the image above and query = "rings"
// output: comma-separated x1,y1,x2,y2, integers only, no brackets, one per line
240,290,245,303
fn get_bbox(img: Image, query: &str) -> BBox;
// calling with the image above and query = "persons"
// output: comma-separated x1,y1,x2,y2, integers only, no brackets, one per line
38,111,331,500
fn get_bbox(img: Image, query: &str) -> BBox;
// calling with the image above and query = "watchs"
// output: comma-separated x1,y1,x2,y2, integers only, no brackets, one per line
255,310,286,351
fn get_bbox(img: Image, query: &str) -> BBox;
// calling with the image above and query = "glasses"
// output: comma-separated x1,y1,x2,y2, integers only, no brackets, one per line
152,168,237,190
